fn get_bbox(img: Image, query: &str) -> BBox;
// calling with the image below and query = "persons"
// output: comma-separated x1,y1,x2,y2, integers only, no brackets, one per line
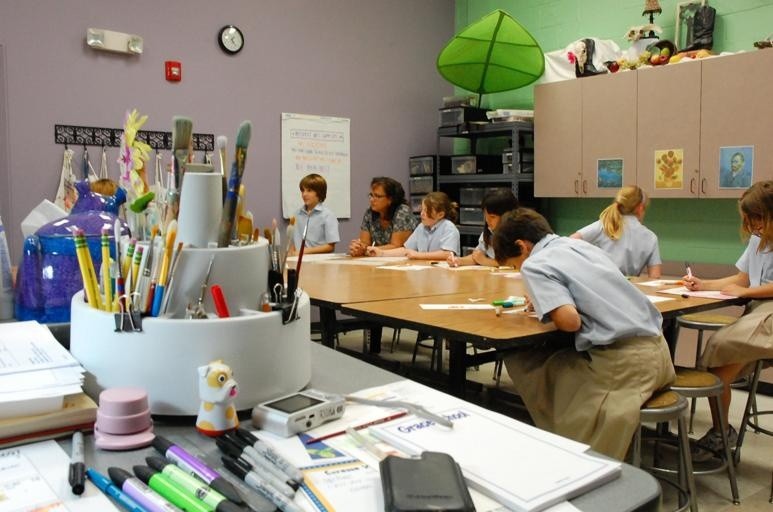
681,180,773,463
568,185,662,279
446,187,521,267
720,152,751,188
366,191,462,260
347,176,420,258
287,173,341,255
491,207,677,463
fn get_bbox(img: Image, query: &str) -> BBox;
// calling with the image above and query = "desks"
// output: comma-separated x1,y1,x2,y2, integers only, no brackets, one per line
0,312,666,512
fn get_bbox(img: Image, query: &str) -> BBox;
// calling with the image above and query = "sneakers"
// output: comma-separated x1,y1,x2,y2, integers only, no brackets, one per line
691,425,739,465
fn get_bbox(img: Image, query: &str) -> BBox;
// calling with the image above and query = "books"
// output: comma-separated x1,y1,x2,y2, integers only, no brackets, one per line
0,319,98,448
249,421,584,511
368,407,622,512
486,108,535,120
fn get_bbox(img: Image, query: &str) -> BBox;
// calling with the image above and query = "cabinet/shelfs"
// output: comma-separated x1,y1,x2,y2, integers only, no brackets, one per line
437,121,534,239
637,47,773,198
534,70,638,199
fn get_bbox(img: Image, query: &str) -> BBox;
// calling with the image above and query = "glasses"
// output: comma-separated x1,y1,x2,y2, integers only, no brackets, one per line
368,192,386,200
747,223,765,238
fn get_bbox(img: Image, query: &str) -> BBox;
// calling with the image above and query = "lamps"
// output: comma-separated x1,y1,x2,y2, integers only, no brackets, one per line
86,28,144,56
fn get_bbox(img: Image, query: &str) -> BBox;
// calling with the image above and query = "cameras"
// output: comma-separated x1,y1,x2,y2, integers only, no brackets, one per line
251,389,346,438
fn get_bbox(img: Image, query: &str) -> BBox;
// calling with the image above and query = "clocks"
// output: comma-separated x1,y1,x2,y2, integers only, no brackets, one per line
219,25,244,54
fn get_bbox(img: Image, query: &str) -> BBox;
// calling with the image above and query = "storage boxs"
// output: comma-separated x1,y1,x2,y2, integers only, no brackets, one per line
409,106,511,227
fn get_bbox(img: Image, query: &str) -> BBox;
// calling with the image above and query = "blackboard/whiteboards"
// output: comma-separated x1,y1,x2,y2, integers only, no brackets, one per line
281,113,353,225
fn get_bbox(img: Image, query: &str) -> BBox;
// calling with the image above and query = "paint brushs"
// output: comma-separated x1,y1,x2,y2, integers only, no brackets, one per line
72,115,314,321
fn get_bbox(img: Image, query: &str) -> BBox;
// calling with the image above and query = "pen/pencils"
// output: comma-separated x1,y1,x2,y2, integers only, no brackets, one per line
685,264,695,286
495,265,514,270
372,241,376,247
661,280,685,286
67,426,311,512
450,251,458,268
492,294,532,317
306,409,409,446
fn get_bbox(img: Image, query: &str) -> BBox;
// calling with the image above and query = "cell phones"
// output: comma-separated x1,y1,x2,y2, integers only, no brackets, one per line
378,451,477,512
